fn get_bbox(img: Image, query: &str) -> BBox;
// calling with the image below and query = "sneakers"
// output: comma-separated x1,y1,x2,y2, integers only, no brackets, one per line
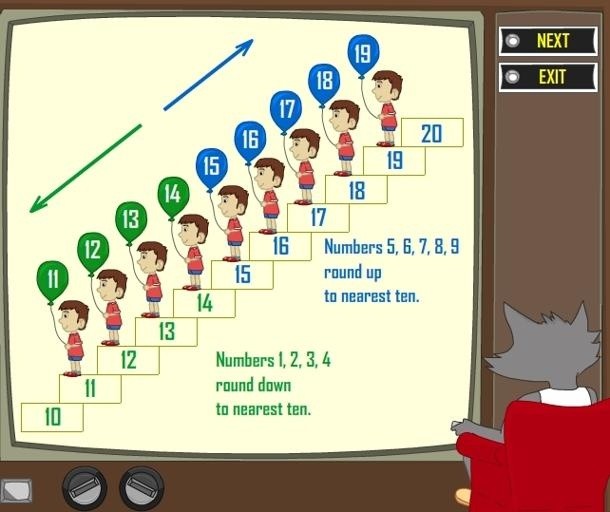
63,371,82,377
182,284,202,291
101,339,121,346
141,312,160,318
258,228,277,234
334,170,352,177
223,256,240,262
376,141,395,148
295,199,312,205
